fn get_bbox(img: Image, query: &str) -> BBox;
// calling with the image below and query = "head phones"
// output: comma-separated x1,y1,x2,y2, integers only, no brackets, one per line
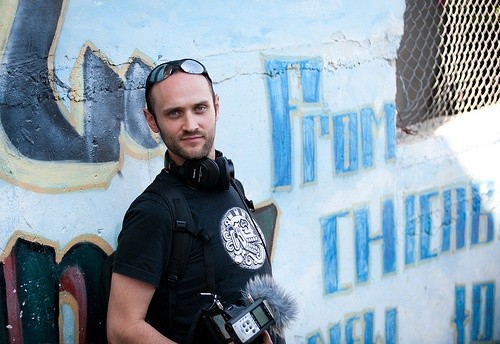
164,148,234,192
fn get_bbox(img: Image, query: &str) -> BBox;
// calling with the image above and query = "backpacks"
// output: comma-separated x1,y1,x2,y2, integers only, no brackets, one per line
98,179,193,344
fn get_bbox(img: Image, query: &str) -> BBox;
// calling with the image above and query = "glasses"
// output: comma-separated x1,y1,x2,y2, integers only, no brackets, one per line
146,59,212,93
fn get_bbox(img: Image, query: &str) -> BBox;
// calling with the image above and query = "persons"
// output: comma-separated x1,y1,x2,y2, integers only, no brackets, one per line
106,59,287,344
